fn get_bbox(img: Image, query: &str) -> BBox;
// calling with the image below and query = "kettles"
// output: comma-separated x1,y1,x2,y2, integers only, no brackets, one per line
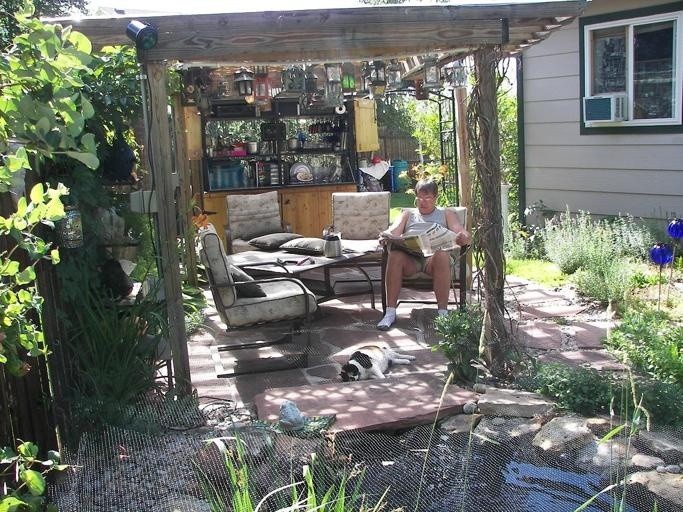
323,236,343,259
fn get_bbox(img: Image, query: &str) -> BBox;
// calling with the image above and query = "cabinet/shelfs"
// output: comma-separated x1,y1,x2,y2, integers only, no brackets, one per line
201,99,357,246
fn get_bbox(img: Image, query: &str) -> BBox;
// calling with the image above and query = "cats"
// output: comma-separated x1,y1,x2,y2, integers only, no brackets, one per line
338,343,417,383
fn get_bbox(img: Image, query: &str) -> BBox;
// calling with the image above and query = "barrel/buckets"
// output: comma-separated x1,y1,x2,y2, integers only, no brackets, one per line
392,159,410,191
322,225,343,258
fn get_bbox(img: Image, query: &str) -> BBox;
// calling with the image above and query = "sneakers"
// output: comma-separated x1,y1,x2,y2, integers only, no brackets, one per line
377,314,396,331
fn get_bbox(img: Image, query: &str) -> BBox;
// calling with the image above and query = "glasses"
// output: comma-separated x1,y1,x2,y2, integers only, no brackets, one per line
416,195,436,202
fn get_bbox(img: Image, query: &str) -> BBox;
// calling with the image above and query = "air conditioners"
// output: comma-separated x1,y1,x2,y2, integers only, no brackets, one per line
583,91,628,123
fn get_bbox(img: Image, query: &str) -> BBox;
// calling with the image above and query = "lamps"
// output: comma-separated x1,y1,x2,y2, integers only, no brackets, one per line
234,68,255,95
365,60,385,84
127,19,160,52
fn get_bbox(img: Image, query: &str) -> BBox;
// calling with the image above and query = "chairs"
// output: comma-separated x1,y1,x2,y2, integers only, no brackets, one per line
324,191,393,297
196,222,318,377
380,207,465,317
225,194,285,256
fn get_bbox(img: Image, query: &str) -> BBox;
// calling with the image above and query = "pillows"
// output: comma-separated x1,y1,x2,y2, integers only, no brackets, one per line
227,263,268,297
247,232,304,250
279,237,326,254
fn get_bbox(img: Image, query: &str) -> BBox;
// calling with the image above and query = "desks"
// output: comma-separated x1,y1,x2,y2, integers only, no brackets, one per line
196,250,375,324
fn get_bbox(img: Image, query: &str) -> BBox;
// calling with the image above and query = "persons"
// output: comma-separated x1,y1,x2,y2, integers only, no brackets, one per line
377,178,473,332
